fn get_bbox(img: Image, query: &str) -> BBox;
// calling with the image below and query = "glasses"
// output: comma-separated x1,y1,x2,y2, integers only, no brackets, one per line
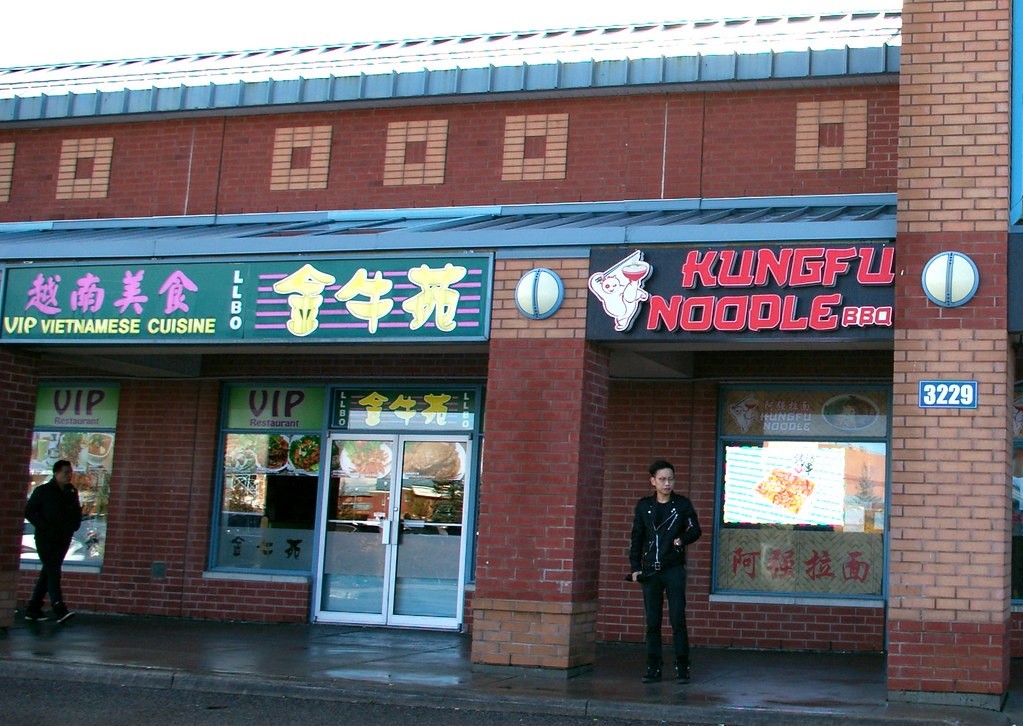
654,476,675,482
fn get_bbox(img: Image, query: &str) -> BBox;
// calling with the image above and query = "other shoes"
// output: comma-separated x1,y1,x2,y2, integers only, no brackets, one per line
642,666,661,683
24,608,50,621
676,667,689,684
56,610,76,623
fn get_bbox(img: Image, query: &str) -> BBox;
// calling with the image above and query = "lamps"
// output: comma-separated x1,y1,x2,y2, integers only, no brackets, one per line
515,266,564,320
922,250,979,309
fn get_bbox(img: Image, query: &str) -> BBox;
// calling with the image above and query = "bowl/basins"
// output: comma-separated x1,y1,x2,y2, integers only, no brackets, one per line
821,394,879,431
87,434,113,465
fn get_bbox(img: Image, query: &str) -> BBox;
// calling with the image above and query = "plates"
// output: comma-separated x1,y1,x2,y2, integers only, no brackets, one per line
403,443,467,481
341,443,392,478
254,434,319,472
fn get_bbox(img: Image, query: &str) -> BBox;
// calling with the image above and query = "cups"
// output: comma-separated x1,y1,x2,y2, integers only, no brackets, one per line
32,436,50,462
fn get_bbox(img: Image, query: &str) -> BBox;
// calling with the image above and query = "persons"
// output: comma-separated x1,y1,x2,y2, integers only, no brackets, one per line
629,461,703,683
25,460,82,622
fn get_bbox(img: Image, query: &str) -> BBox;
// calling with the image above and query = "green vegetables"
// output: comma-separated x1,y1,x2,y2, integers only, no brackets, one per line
237,432,320,469
59,432,103,455
343,440,383,455
